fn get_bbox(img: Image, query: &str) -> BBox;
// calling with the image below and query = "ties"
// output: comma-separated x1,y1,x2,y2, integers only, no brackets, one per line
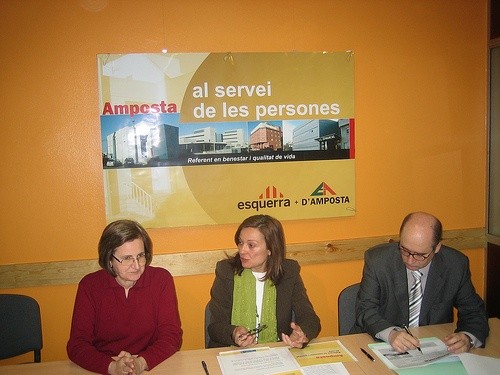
409,270,423,327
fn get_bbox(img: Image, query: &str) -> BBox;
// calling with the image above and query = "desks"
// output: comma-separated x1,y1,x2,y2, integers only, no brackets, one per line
0,317,500,375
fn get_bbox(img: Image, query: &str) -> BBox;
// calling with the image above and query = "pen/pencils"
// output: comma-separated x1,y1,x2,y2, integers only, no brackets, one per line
404,324,423,355
359,346,375,363
201,360,210,375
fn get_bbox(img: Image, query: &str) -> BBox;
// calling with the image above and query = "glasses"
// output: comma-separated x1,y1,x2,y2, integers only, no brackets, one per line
111,250,148,265
398,241,435,261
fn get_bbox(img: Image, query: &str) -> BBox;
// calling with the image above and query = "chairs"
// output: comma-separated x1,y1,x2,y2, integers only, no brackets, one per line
338,283,362,336
0,293,43,365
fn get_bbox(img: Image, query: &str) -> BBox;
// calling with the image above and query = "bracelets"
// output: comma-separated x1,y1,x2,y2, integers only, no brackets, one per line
466,334,473,348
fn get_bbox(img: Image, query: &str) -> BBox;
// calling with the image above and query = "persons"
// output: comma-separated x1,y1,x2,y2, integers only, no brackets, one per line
355,211,489,355
67,220,182,375
207,214,322,349
